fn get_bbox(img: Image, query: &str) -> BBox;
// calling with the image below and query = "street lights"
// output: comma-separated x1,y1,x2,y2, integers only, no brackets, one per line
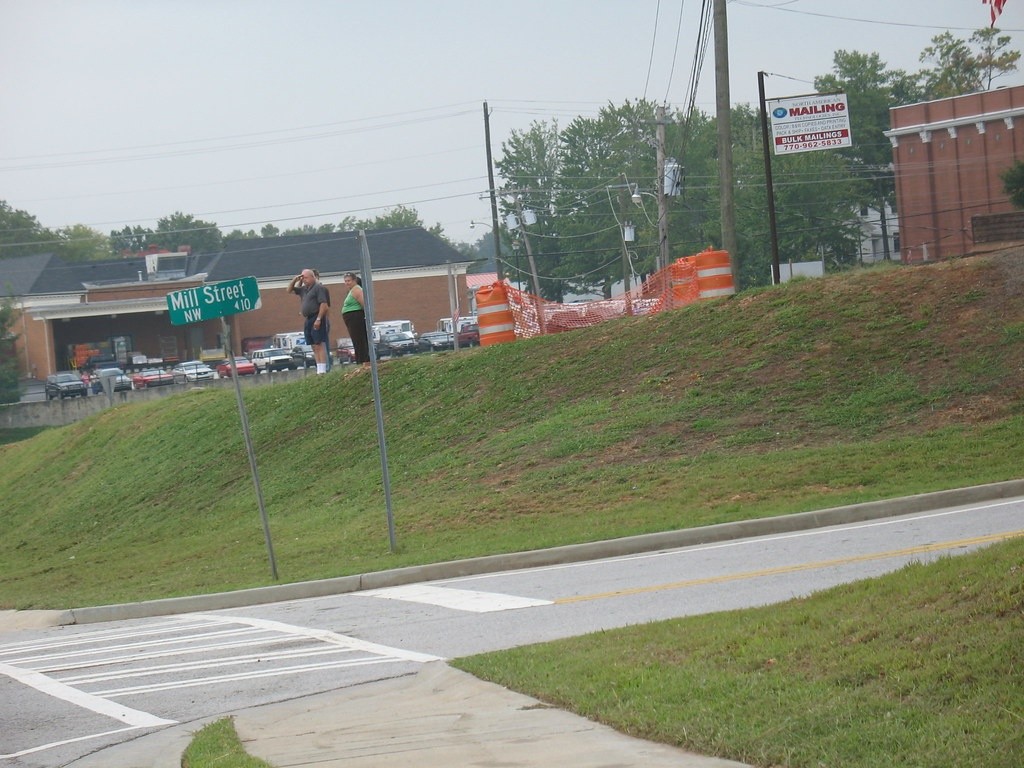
470,220,502,281
511,241,524,313
631,183,673,311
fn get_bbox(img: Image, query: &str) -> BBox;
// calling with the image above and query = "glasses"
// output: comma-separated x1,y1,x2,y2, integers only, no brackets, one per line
344,273,350,276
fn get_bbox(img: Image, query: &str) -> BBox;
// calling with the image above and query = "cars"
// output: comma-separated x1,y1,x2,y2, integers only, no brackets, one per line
457,323,479,348
252,348,295,373
173,361,214,384
90,368,132,394
376,333,420,359
46,374,88,399
336,341,358,364
217,357,255,378
132,370,174,389
419,332,454,352
85,354,125,373
291,345,333,368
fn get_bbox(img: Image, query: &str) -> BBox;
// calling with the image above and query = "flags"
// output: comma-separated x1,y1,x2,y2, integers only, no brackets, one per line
453,307,460,321
981,0,1008,26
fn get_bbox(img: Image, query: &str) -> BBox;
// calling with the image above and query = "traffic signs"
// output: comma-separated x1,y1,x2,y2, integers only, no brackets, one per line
167,276,263,326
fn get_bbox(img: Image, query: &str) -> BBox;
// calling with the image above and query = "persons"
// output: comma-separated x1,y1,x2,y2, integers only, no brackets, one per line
286,269,331,375
341,272,370,365
81,371,97,389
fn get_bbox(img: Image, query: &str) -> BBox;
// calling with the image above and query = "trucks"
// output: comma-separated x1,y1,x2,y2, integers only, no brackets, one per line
273,332,306,349
372,320,414,341
437,316,477,332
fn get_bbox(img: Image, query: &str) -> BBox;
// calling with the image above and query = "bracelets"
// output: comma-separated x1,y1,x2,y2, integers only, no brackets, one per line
317,318,321,320
